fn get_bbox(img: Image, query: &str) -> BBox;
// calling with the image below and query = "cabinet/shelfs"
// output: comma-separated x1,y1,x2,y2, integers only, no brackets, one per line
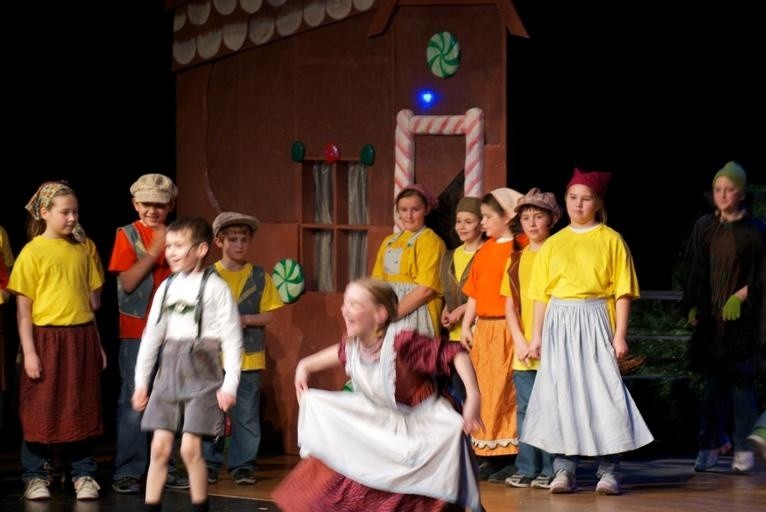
608,290,708,401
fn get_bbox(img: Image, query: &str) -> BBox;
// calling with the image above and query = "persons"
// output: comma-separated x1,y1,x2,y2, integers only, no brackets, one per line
270,277,487,512
371,183,447,338
460,186,529,483
130,215,244,512
202,211,285,484
441,196,484,344
518,167,654,494
107,173,189,494
500,186,562,490
678,161,766,475
6,180,107,501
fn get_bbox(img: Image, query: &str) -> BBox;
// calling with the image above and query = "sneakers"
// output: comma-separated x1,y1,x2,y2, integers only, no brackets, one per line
74,478,102,499
113,477,139,493
232,469,256,484
207,469,219,482
596,473,622,494
479,461,576,492
163,470,191,488
732,452,754,474
23,478,52,500
695,441,732,470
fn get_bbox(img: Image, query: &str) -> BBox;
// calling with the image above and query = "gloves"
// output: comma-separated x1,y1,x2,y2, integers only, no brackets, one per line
688,310,696,326
722,294,743,322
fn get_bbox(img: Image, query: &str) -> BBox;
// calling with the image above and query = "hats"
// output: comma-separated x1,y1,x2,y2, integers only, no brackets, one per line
456,196,482,215
213,211,260,237
129,174,178,204
514,188,562,226
713,162,747,193
